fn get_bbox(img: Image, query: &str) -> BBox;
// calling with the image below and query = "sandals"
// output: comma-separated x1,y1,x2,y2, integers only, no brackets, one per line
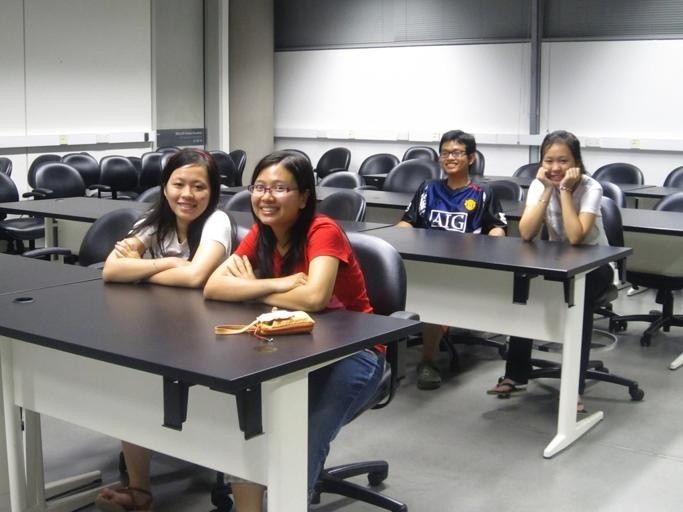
97,485,152,511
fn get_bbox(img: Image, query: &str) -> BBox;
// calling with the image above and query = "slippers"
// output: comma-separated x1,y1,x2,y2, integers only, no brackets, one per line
576,402,586,414
486,382,526,394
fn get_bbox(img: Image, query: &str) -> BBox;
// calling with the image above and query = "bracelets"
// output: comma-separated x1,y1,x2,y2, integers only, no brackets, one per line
559,186,573,191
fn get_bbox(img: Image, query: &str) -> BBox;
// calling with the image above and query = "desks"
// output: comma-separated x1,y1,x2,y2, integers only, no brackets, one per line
227,209,395,239
315,188,416,224
505,207,682,370
1,251,102,501
0,196,154,259
363,173,387,190
220,185,354,204
624,185,682,209
0,279,425,512
476,175,656,200
357,227,633,458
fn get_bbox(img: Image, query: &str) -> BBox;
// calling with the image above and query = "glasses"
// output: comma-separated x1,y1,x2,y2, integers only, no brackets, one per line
440,150,466,158
247,184,296,195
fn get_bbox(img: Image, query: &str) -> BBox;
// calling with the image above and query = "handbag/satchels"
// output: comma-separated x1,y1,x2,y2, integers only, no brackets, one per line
214,308,314,337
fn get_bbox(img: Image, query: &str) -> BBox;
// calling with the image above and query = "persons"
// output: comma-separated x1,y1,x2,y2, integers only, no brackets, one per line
486,131,617,413
203,150,387,512
96,146,231,512
396,130,506,389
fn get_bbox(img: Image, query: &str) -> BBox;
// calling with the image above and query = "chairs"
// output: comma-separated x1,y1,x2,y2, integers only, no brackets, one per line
610,190,683,347
383,158,441,193
592,163,643,186
0,172,57,251
90,157,141,200
512,162,541,178
161,152,176,172
31,161,111,199
663,166,683,188
155,146,182,153
402,146,439,162
27,154,62,188
498,195,644,401
358,154,400,190
225,190,251,211
22,209,144,269
135,185,161,203
408,327,508,376
470,150,485,175
139,152,163,194
0,157,13,177
313,147,351,185
210,231,408,512
485,179,524,202
598,180,627,209
118,206,250,484
208,150,236,187
99,155,138,199
317,190,366,222
318,171,366,191
229,150,247,187
61,153,100,189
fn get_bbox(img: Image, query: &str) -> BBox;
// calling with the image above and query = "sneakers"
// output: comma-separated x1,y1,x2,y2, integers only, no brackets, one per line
417,358,441,389
